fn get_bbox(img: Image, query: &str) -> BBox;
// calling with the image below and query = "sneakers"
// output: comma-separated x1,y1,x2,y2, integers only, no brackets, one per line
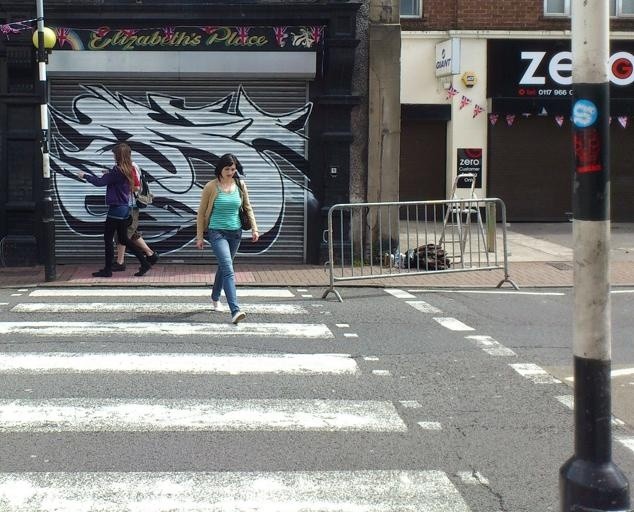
232,311,247,324
210,293,225,312
92,270,112,277
134,251,159,276
104,261,126,271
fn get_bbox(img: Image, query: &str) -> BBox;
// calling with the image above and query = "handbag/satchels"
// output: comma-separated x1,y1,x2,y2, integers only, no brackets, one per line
240,208,251,230
135,176,154,208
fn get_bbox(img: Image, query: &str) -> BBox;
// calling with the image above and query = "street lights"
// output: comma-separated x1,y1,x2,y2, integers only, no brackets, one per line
28,22,60,283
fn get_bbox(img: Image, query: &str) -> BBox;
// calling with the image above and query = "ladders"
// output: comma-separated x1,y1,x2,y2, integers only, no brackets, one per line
439,174,492,270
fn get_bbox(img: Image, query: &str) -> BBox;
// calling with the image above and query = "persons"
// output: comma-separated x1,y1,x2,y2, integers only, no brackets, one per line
109,145,159,272
77,143,151,277
196,152,259,324
